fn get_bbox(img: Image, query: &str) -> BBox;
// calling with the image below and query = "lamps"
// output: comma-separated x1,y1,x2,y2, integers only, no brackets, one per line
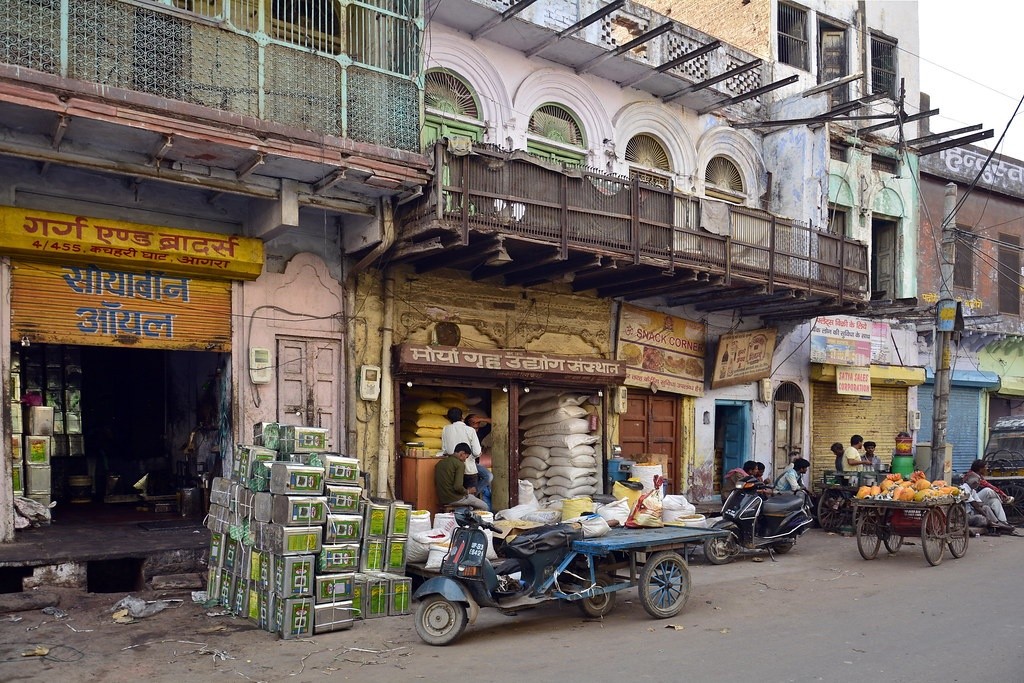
484,233,513,266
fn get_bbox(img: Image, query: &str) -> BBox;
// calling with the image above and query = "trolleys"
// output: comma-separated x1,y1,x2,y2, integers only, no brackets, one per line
851,494,970,566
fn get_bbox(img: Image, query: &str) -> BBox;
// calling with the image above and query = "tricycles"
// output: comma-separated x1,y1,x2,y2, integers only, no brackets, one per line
410,506,731,645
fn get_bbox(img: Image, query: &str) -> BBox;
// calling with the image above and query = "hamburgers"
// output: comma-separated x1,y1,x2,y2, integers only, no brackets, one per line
619,342,643,364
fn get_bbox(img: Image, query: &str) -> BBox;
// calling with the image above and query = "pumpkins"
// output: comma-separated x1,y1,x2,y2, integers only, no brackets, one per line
856,470,962,501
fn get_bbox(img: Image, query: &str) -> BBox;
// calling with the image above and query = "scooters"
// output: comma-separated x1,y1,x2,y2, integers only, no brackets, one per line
704,472,813,564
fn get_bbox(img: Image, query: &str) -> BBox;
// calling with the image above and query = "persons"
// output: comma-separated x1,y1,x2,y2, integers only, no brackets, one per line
721,460,758,506
461,413,495,498
959,471,1016,532
434,443,490,511
890,431,919,471
859,440,881,471
831,441,846,472
754,462,772,500
775,458,818,500
840,434,873,472
962,459,1008,503
441,408,483,487
